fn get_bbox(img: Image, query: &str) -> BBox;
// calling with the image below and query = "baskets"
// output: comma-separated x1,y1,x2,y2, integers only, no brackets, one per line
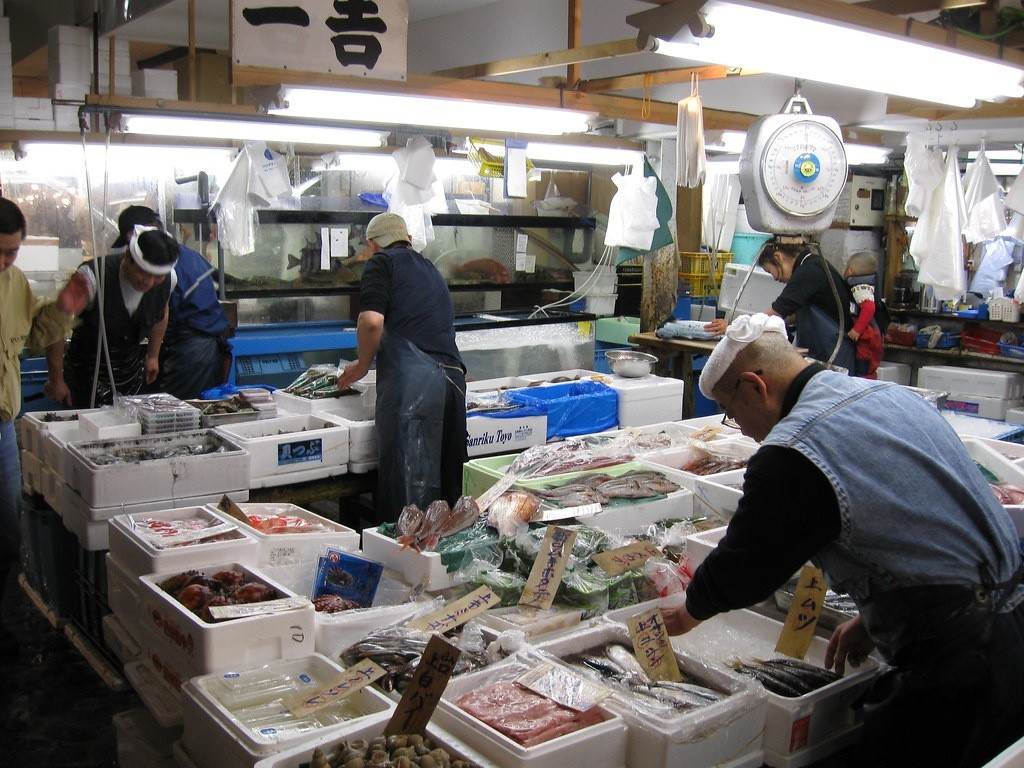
883,298,1024,358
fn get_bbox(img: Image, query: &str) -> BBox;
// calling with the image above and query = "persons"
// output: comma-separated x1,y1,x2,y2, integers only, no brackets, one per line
704,238,856,376
659,313,1024,768
843,252,890,381
111,204,228,399
338,213,467,528
0,197,34,660
45,226,181,410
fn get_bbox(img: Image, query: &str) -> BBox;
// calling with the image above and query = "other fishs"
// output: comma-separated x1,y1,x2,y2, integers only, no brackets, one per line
337,421,854,695
445,256,510,286
286,224,369,283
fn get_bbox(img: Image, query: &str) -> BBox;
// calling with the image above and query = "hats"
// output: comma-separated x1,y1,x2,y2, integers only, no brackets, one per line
111,206,157,248
365,212,413,248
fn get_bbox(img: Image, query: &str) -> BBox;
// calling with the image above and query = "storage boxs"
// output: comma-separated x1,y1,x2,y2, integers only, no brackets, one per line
0,14,179,134
19,171,1024,768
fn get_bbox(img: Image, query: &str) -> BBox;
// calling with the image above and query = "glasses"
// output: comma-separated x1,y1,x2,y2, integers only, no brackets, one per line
720,370,763,429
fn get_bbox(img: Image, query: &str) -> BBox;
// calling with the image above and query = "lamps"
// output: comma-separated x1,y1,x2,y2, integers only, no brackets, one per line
12,0,1023,189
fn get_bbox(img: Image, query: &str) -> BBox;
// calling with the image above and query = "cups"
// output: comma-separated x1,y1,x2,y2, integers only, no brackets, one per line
569,296,586,312
894,287,914,302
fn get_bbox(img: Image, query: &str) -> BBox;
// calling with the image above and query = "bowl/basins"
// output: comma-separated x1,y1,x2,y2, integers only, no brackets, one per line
357,193,388,211
605,351,659,377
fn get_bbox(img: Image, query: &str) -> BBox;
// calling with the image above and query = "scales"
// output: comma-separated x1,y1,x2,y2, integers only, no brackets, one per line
722,79,849,444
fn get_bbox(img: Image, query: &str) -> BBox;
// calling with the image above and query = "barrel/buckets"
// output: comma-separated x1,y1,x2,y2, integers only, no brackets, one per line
730,233,774,264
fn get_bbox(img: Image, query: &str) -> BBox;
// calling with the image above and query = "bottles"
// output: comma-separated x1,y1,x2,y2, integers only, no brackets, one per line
968,305,973,310
